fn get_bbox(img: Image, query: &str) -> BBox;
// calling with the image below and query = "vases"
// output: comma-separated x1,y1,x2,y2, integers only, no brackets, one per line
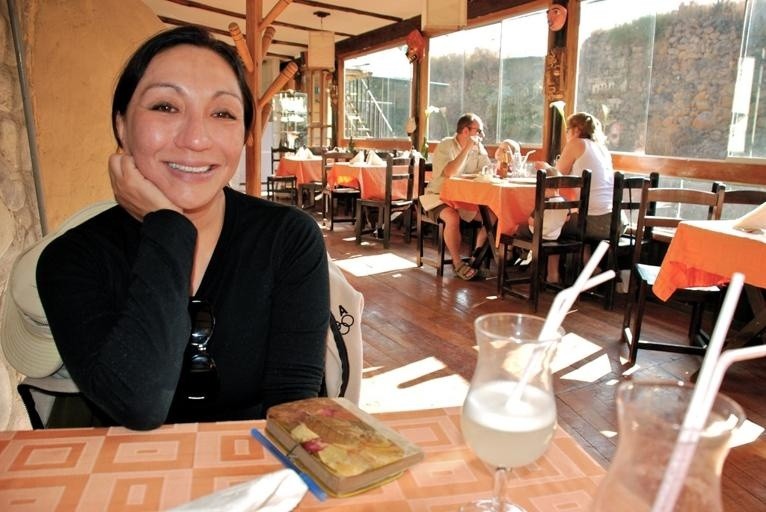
349,136,353,153
422,137,428,159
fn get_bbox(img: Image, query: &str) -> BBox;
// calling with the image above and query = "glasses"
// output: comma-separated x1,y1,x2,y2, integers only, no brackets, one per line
180,296,221,401
469,128,482,134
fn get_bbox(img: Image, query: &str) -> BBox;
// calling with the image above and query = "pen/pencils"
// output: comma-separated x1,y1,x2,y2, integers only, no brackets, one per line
251,428,326,501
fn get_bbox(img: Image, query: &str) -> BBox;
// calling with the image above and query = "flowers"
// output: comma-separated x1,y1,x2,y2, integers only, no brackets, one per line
440,107,449,137
549,99,566,131
348,116,359,136
423,105,440,136
601,103,610,133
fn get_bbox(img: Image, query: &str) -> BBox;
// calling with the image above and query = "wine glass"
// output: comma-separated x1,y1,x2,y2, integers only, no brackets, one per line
460,310,565,512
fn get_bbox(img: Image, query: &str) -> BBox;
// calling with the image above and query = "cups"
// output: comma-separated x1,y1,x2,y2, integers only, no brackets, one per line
480,148,533,184
592,377,747,512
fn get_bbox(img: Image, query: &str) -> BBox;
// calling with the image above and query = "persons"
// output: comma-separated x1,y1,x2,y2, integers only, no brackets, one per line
493,139,521,164
504,167,572,273
30,23,333,431
546,4,568,32
546,111,630,285
425,111,498,281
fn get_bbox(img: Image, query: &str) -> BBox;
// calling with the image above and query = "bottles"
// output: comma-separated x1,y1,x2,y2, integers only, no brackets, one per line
420,138,429,162
556,155,561,169
348,136,355,154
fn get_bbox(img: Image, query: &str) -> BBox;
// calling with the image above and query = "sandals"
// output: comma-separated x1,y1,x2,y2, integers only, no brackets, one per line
453,262,478,280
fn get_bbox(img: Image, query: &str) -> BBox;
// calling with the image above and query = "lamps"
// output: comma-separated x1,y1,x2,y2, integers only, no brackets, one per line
308,10,336,72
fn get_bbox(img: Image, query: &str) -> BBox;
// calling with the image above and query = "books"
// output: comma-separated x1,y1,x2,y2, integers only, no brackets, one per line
263,393,426,496
